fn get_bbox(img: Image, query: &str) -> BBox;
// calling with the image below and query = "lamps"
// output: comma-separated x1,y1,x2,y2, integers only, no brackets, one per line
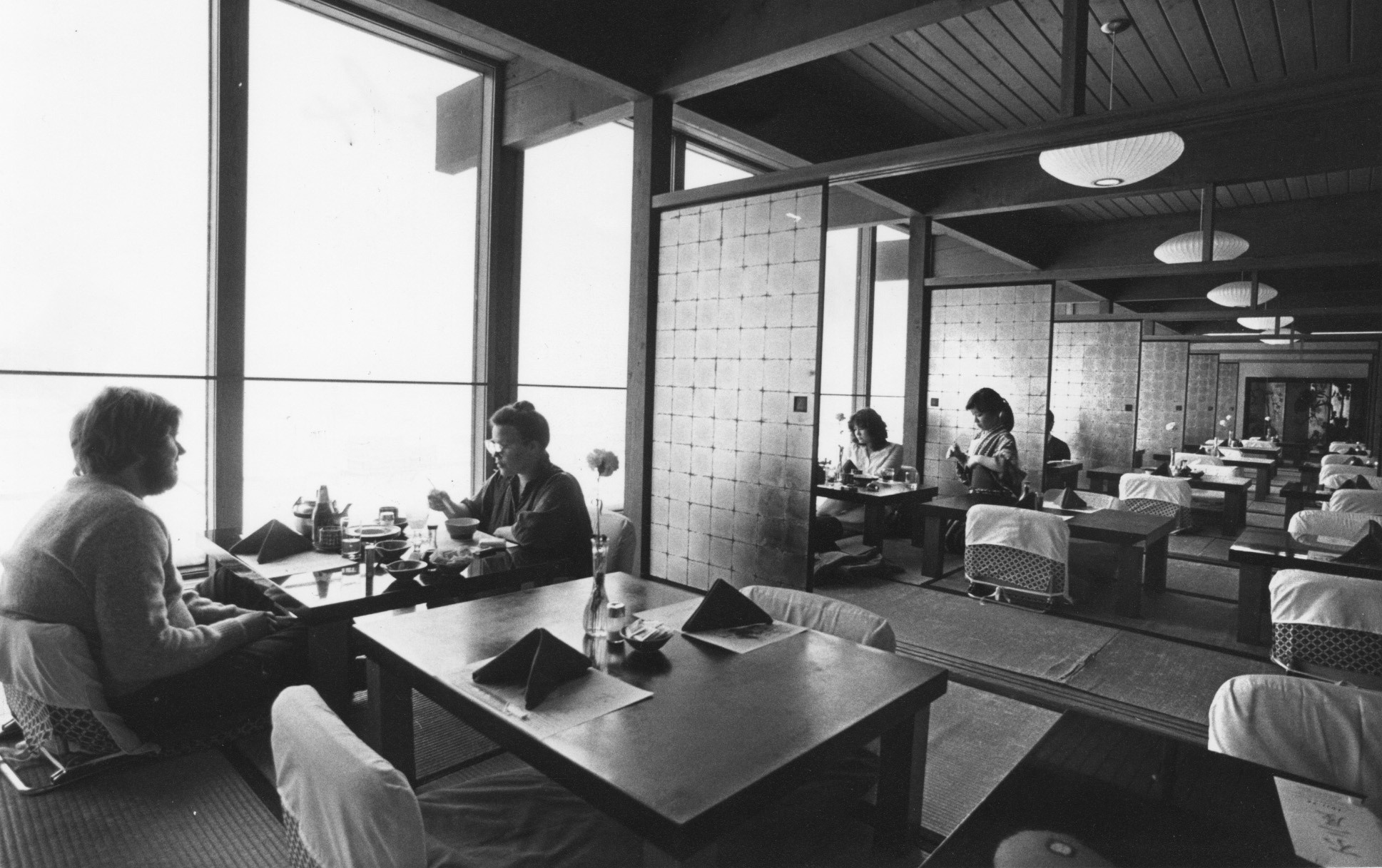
1039,17,1303,347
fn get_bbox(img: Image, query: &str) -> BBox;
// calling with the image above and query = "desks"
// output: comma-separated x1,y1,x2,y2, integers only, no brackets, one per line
349,572,951,868
810,474,937,559
1279,480,1334,523
1086,465,1253,537
1216,454,1276,502
917,492,1175,615
200,527,575,721
917,708,1382,868
1232,524,1380,647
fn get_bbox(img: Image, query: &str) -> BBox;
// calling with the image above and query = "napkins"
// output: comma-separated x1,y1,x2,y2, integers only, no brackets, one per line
681,578,773,633
1150,461,1172,477
1051,486,1088,509
229,517,316,566
1337,474,1374,489
472,627,592,713
829,461,858,481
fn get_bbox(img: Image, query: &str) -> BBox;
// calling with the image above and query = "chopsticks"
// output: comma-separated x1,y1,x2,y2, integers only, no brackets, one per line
427,477,455,515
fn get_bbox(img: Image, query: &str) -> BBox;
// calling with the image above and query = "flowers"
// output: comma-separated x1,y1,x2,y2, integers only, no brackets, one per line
587,447,618,632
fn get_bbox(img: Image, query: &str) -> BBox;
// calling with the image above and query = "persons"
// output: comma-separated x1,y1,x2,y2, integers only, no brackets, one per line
1301,430,1333,462
815,387,1026,558
1218,427,1243,447
429,401,594,601
1258,427,1280,442
2,387,353,728
1043,410,1070,493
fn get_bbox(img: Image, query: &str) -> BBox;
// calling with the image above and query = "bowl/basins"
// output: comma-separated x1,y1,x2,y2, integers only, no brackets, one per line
618,625,674,652
375,540,411,561
319,526,342,551
445,518,480,540
376,517,408,531
429,555,472,575
853,474,879,487
385,560,428,581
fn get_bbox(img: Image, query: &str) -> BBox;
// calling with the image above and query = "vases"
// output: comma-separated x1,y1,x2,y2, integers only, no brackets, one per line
582,532,613,638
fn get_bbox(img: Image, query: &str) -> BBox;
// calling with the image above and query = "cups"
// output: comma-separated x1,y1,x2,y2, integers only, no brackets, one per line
340,517,362,560
379,511,395,526
881,467,895,489
905,472,918,491
379,506,399,523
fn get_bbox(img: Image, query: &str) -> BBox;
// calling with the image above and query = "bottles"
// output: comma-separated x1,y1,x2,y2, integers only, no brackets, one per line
418,524,438,578
606,601,625,643
311,485,332,549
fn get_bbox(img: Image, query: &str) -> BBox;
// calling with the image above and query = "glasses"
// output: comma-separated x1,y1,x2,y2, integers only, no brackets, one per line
484,438,530,457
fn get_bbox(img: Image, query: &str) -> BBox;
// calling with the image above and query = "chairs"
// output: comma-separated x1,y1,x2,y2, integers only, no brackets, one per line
0,444,1381,868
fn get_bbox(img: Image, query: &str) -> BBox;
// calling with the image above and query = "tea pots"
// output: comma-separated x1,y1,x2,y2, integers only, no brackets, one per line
292,496,352,537
